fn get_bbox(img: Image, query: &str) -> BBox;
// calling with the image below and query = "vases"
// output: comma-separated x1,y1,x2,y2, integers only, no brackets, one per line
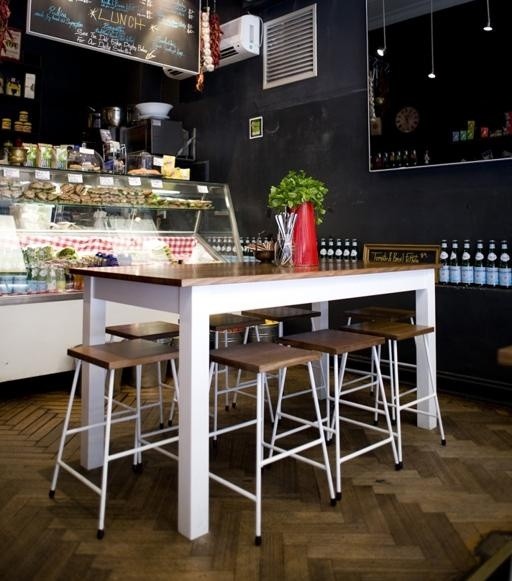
289,205,320,266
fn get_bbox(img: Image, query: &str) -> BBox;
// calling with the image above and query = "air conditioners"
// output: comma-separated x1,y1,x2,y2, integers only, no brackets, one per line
162,15,263,81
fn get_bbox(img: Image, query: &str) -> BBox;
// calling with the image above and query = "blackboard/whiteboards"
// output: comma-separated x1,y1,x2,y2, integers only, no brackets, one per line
27,0,201,75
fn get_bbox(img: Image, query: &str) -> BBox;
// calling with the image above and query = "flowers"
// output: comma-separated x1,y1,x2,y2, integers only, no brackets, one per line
267,170,329,224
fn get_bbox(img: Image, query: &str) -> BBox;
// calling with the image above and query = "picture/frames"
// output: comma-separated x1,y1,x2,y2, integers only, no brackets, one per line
362,243,440,265
249,116,263,140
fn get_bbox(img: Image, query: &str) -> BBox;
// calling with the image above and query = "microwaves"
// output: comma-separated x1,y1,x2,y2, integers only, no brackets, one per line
120,118,184,156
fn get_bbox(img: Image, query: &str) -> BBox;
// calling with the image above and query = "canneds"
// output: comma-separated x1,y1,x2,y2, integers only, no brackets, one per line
23,123,31,132
3,118,11,129
20,111,28,122
15,121,23,132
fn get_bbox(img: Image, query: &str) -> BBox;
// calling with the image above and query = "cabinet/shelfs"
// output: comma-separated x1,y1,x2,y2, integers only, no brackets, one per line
0,164,243,380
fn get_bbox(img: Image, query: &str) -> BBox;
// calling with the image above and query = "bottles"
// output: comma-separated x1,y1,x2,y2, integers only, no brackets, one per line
461,240,473,285
207,237,212,246
450,240,461,285
486,240,499,287
335,239,343,259
222,237,227,254
25,255,107,293
320,239,327,258
474,239,486,286
212,237,217,250
258,237,262,244
343,239,351,260
217,237,222,254
227,237,231,255
439,240,450,284
327,239,335,258
373,148,417,168
245,237,249,243
350,239,358,260
424,149,430,162
240,237,244,243
263,236,269,244
499,240,512,288
252,237,256,244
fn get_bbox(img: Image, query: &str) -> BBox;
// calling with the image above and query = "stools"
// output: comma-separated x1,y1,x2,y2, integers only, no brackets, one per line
275,330,398,499
344,319,446,468
336,306,417,424
233,308,329,413
210,314,274,422
104,321,179,463
208,342,338,545
47,337,186,538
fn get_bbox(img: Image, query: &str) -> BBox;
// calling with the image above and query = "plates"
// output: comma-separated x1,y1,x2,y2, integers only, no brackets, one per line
137,115,170,120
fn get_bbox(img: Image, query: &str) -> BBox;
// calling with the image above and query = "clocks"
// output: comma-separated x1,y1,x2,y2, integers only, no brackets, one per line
395,107,419,135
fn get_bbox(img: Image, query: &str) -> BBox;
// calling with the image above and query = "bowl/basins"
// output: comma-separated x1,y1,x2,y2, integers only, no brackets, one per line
253,251,275,264
135,102,173,115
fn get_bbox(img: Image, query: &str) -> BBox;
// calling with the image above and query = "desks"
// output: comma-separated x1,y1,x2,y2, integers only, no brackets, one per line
69,261,443,541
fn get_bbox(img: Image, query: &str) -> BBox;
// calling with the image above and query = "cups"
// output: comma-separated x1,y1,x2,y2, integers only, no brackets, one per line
274,212,298,269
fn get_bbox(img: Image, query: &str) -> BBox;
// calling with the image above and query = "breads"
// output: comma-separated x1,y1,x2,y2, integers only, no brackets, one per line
9,177,22,190
58,184,145,204
0,177,9,190
35,192,47,203
24,190,35,201
0,190,11,200
47,194,57,202
43,182,56,193
12,191,24,202
29,182,42,192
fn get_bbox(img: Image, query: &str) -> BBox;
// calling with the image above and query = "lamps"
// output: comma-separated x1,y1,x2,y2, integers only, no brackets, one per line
376,1,387,56
428,1,436,79
483,0,492,31
